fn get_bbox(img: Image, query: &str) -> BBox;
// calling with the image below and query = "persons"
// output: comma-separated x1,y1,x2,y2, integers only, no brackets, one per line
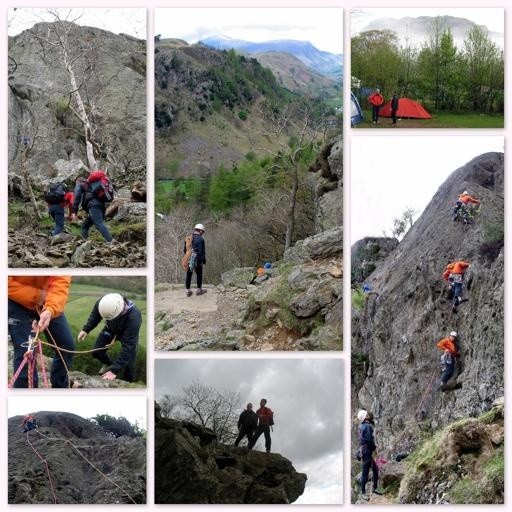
448,188,482,227
430,330,465,392
20,415,38,434
8,276,143,388
438,257,476,318
180,223,207,297
354,409,387,501
231,399,274,453
43,171,115,241
369,88,398,126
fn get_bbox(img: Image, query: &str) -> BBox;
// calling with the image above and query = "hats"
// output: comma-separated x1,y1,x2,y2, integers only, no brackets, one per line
194,224,205,233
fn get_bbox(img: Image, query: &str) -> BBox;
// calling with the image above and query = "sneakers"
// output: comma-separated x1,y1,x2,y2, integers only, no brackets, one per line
197,287,207,295
361,494,369,501
372,489,382,495
187,289,193,297
98,364,113,374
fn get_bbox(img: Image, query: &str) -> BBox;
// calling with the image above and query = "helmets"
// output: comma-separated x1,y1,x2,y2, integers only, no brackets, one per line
98,293,124,320
450,331,458,337
358,410,368,423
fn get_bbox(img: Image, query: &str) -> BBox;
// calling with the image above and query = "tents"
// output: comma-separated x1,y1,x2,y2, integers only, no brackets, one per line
378,98,432,119
351,91,363,125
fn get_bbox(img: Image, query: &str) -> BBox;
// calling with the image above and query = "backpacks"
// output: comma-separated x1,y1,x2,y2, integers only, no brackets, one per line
44,182,70,204
88,171,114,203
